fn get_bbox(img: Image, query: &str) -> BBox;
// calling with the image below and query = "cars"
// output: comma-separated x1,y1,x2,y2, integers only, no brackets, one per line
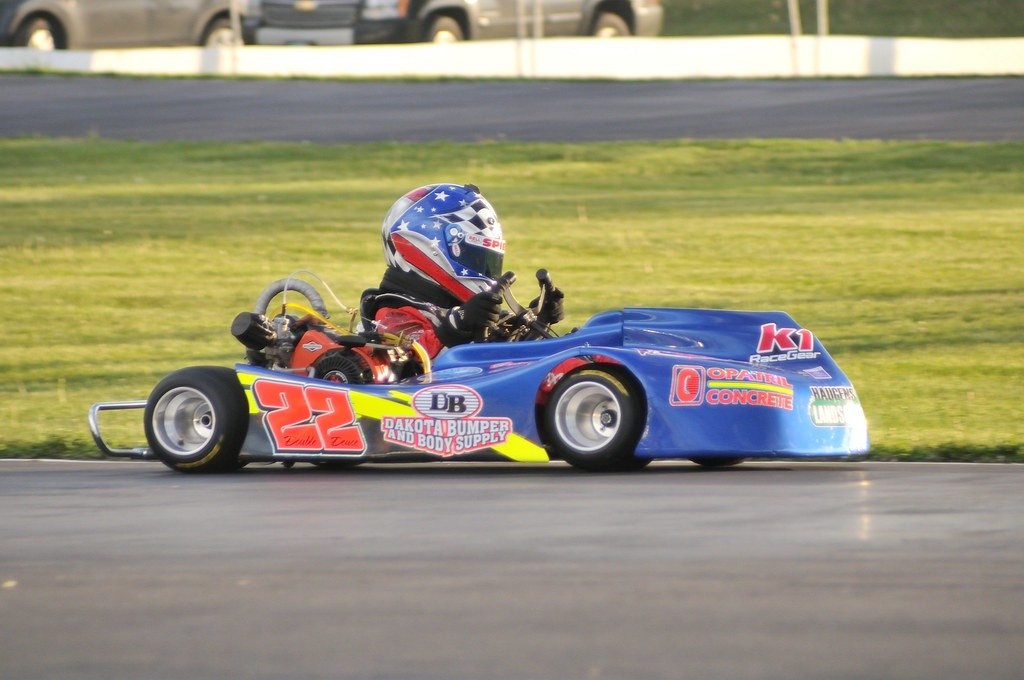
0,1,237,51
240,0,663,45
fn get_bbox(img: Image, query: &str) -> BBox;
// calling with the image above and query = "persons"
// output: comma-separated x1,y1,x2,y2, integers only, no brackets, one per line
356,183,565,363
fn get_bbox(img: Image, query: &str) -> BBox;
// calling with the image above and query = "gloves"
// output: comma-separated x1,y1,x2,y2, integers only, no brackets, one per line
435,293,503,349
527,287,564,326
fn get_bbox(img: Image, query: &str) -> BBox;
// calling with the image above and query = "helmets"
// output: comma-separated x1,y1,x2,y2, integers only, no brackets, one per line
382,183,506,304
